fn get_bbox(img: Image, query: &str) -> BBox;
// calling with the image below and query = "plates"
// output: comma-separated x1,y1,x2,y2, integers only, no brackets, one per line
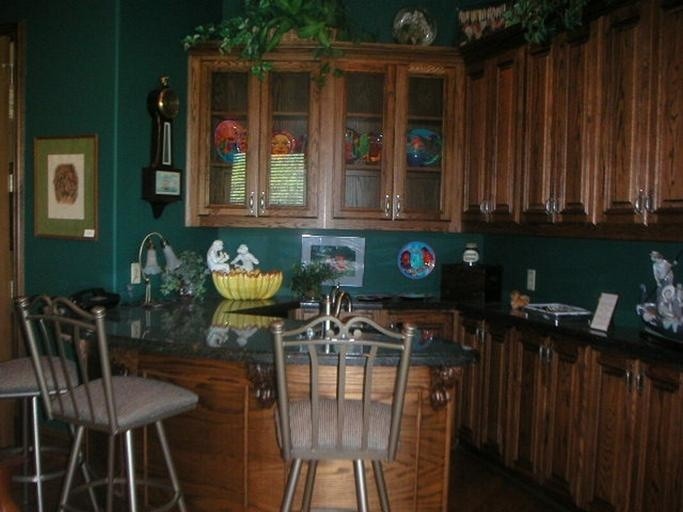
215,118,442,166
397,241,437,281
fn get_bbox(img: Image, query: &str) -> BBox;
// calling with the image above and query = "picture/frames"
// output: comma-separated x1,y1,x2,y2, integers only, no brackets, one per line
32,134,98,240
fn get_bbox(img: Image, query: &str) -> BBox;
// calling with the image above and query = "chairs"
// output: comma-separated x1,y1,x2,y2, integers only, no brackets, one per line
13,293,200,511
270,312,418,512
3,351,97,510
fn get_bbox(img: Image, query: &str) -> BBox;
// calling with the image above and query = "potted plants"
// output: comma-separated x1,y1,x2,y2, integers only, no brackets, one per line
162,251,209,305
288,261,339,309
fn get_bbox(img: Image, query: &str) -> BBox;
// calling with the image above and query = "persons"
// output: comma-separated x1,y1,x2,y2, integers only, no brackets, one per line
207,240,259,274
650,250,683,333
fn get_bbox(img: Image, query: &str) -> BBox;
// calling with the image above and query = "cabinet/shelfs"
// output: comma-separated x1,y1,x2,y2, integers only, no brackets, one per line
461,21,520,222
597,0,682,230
519,2,597,225
326,42,469,228
187,39,331,233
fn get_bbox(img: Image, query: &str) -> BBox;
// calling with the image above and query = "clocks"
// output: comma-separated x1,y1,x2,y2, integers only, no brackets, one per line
140,75,184,221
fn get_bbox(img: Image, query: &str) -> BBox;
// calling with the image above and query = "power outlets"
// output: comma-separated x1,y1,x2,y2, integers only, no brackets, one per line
130,262,142,286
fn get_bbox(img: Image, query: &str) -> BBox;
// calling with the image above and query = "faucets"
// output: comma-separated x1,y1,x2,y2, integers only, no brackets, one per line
322,290,352,337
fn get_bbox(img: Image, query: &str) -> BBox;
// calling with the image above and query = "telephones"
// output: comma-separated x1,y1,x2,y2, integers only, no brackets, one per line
70,288,121,312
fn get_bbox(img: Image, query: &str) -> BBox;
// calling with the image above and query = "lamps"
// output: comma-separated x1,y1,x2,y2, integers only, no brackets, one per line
137,230,182,310
